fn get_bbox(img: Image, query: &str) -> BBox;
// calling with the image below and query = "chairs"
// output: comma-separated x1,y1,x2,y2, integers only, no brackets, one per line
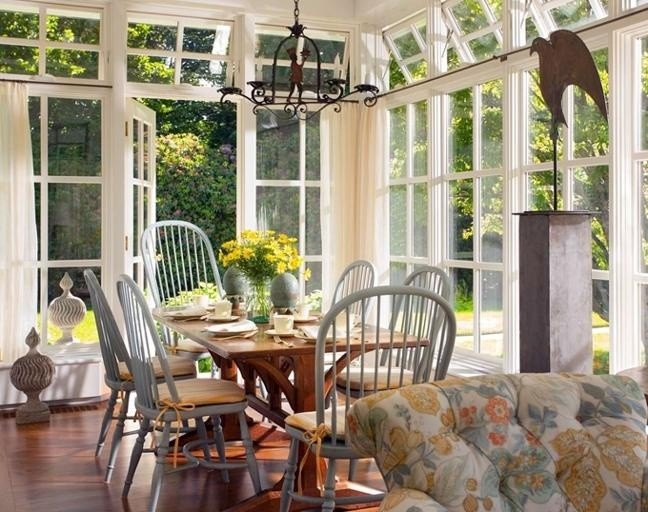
345,375,648,510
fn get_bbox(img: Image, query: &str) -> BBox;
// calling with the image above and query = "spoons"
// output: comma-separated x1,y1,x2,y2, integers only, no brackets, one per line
211,330,259,341
174,315,207,323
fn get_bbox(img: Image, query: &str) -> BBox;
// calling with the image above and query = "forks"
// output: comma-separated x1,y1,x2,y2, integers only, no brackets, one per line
273,336,294,347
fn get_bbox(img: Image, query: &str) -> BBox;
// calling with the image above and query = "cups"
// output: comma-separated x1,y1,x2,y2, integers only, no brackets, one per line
215,302,232,318
297,304,310,317
273,315,294,333
193,295,209,308
336,313,359,330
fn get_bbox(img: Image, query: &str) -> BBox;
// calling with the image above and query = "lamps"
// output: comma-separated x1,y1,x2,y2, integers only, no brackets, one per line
216,0,378,120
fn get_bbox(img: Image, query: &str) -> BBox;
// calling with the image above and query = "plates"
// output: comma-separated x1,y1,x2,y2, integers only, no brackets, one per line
294,316,318,323
207,323,258,336
163,310,210,320
207,306,215,310
294,331,354,344
351,327,361,333
206,315,240,322
264,329,298,337
